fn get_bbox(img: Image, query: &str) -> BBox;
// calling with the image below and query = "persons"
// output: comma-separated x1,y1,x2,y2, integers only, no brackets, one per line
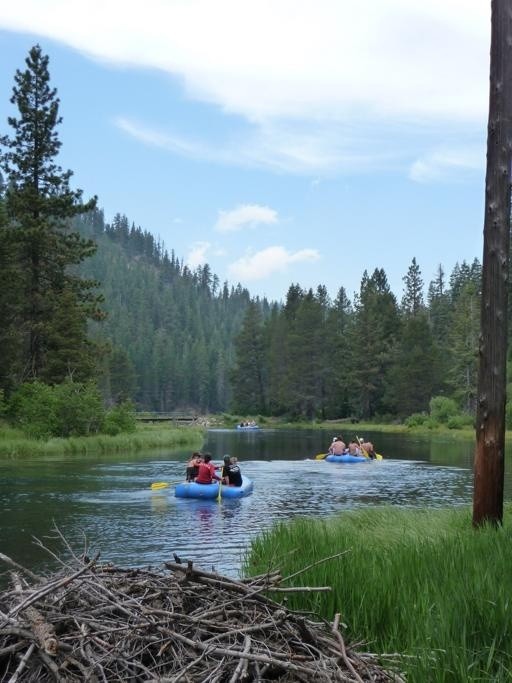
239,417,257,428
221,454,242,488
360,437,374,457
347,439,360,457
328,433,346,456
183,450,204,482
193,453,224,485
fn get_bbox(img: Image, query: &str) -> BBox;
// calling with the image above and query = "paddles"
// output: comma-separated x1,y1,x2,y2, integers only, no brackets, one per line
152,480,190,490
356,435,369,457
374,453,383,460
316,454,330,459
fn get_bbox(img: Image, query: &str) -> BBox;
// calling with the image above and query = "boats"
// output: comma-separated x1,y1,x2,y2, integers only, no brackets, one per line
174,470,254,499
236,423,259,429
325,447,371,463
